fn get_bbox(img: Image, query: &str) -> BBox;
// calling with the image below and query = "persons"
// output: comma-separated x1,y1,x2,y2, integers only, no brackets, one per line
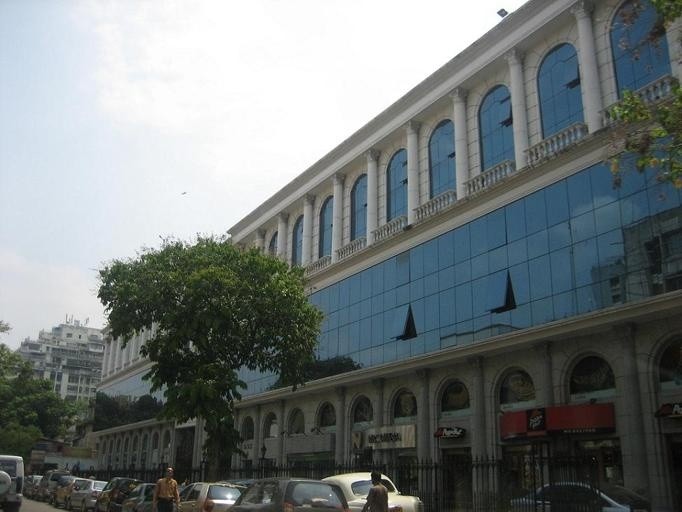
152,466,182,512
361,470,389,512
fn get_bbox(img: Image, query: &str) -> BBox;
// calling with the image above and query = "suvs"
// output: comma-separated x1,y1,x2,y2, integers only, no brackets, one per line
0,454,24,512
95,477,143,511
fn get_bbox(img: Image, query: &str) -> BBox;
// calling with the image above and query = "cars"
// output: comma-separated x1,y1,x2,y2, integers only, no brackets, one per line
121,482,156,511
509,481,652,511
175,482,241,511
323,471,424,510
227,477,348,511
65,479,109,512
24,469,90,508
